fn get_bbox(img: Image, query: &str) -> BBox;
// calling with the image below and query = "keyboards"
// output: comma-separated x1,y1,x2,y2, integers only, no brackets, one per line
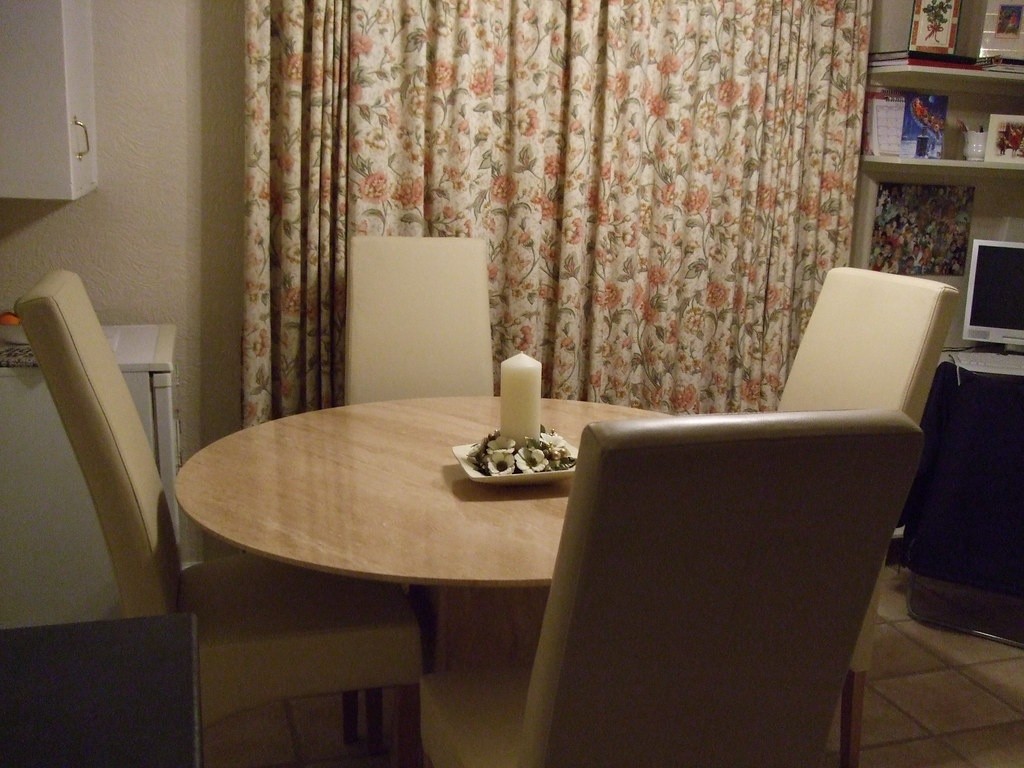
949,352,1024,376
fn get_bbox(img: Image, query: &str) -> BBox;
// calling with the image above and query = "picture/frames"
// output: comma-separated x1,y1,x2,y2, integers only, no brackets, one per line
984,113,1024,163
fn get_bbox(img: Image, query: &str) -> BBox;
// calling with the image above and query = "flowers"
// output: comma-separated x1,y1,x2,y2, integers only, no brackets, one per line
466,425,578,477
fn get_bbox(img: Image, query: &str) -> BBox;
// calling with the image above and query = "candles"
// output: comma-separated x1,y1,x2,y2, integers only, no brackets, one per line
500,350,542,450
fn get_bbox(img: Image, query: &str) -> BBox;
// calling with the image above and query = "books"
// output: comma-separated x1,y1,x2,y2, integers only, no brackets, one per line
866,51,1024,74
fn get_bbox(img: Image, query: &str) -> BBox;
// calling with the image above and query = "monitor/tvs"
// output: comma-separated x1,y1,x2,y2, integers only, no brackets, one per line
962,238,1024,355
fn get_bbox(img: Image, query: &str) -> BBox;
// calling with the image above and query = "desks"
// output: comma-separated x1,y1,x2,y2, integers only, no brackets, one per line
894,362,1024,650
176,396,677,768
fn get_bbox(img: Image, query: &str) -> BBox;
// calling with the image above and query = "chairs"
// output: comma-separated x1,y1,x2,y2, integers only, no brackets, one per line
778,266,961,768
418,408,925,768
16,238,489,768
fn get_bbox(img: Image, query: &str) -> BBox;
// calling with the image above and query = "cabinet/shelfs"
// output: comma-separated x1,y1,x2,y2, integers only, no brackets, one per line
0,0,99,201
857,64,1024,182
0,323,180,631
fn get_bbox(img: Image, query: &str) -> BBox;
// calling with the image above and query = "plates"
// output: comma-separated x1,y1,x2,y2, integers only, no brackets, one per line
452,433,579,484
